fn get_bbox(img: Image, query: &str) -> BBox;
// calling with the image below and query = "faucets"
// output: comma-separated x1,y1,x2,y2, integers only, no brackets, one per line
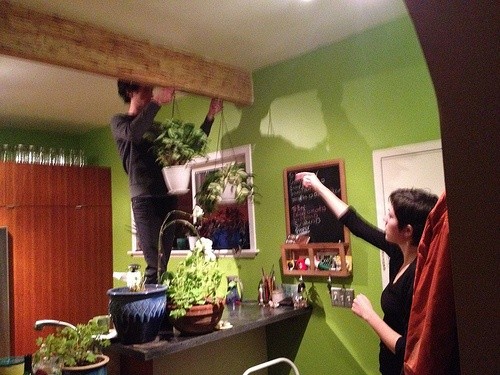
33,319,81,335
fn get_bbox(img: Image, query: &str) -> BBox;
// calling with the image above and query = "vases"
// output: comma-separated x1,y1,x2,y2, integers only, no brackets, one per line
107,285,169,345
167,302,224,334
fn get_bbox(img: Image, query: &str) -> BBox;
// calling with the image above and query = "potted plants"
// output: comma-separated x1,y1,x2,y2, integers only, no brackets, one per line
32,316,111,375
142,119,210,191
194,161,263,212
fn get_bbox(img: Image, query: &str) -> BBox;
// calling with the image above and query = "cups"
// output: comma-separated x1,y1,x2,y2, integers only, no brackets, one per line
256,286,309,309
295,235,311,244
87,315,110,333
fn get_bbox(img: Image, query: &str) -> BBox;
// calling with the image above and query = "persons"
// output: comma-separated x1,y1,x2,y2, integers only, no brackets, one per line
296,172,439,375
111,78,223,284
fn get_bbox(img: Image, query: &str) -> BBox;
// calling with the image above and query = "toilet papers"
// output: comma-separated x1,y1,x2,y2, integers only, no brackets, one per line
126,270,141,287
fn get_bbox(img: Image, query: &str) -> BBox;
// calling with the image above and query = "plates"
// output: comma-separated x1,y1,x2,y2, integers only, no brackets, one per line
92,329,118,340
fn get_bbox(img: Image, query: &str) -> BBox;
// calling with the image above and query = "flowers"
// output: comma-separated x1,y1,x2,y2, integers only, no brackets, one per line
156,205,237,319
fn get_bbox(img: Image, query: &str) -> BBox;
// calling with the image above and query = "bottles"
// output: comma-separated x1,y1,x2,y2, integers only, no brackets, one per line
293,276,306,294
225,276,240,304
126,263,143,286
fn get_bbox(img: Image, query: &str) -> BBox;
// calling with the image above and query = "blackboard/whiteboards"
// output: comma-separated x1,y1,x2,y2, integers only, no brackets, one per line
282,157,350,248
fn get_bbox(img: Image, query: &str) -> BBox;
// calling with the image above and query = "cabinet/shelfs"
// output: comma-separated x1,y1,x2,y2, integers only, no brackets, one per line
0,161,113,355
281,242,353,277
